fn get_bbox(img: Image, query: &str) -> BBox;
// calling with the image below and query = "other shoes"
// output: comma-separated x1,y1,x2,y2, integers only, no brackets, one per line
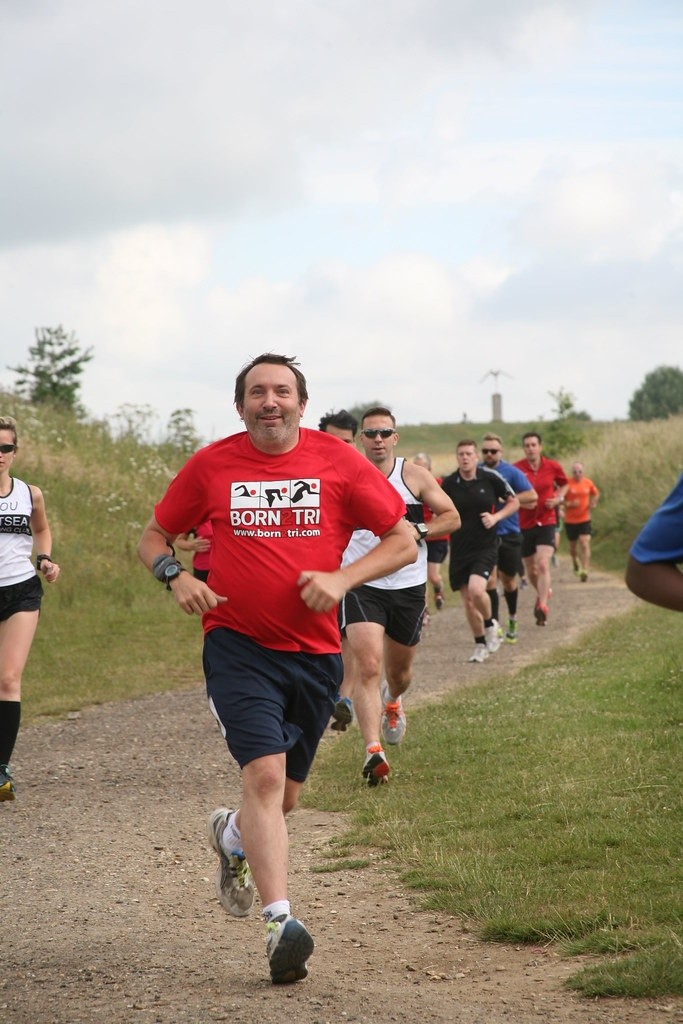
572,559,581,576
552,554,556,567
580,571,587,581
521,574,527,585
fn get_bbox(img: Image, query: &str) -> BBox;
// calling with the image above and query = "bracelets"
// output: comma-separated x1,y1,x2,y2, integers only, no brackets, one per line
558,495,564,501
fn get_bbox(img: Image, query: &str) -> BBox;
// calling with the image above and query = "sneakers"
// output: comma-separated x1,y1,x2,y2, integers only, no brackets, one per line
484,619,503,653
0,764,16,801
362,739,389,787
533,596,547,625
421,607,430,626
267,912,314,985
468,643,489,663
381,679,406,744
331,687,353,731
209,806,255,917
435,582,445,609
506,613,517,644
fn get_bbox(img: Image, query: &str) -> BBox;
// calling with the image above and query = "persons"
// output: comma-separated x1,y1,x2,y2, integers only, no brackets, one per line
137,354,417,984
338,408,461,786
320,410,358,732
514,432,571,625
1,417,61,801
626,473,683,613
177,520,213,582
440,440,520,661
413,454,450,626
477,434,538,643
552,463,600,580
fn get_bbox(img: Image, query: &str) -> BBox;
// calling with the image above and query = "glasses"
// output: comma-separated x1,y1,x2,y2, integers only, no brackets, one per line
482,449,501,455
0,445,16,453
343,440,352,444
361,429,396,438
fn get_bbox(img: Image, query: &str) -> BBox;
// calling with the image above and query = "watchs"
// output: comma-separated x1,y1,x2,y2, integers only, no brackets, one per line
414,522,428,547
163,564,188,591
37,554,52,571
